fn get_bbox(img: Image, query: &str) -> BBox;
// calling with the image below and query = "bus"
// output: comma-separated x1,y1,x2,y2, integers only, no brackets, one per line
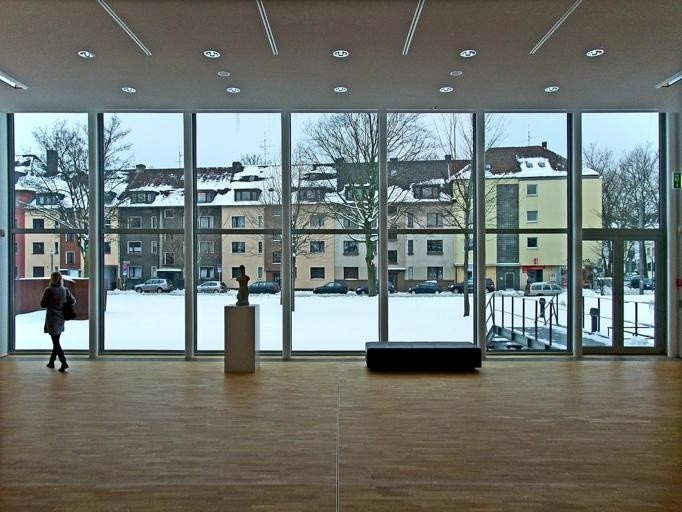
562,269,593,288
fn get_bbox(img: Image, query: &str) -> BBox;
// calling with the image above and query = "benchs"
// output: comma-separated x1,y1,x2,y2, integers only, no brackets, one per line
365,342,483,373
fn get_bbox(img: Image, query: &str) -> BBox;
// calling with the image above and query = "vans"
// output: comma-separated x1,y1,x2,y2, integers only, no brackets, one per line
595,276,612,294
524,282,564,297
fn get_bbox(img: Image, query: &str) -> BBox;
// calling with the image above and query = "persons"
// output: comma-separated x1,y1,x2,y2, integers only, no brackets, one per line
37,270,78,374
234,264,251,306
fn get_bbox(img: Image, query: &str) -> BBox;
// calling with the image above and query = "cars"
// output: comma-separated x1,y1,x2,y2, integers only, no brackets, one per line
313,281,347,294
408,280,441,294
631,276,654,290
249,281,280,294
197,281,227,293
355,280,394,295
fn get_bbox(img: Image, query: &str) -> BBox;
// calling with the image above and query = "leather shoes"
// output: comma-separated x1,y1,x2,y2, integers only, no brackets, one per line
47,363,54,368
59,364,68,372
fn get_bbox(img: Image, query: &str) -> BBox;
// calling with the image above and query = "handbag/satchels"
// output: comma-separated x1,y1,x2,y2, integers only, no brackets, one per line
63,302,77,320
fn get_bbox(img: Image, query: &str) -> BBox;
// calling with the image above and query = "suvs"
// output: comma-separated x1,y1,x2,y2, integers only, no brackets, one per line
134,278,174,293
448,277,494,293
